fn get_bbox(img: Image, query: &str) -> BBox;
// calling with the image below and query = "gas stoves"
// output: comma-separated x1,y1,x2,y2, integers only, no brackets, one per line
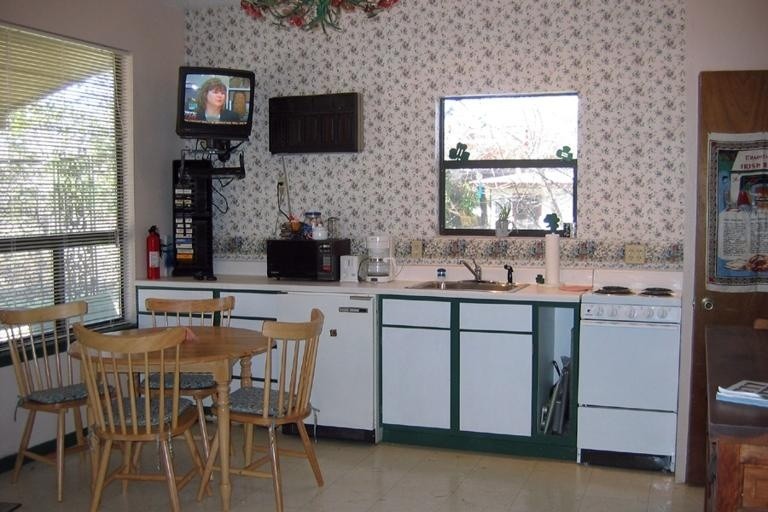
580,285,681,324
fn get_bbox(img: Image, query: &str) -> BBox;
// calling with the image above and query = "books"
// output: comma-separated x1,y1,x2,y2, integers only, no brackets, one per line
715,379,768,408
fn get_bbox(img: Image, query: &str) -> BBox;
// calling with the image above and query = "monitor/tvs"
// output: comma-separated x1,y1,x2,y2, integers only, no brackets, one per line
176,65,256,142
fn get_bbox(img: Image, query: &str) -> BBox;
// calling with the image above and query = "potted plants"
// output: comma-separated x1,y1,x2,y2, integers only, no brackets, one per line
496,201,515,238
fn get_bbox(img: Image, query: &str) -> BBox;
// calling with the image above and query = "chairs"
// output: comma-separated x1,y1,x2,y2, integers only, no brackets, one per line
1,300,120,504
138,294,236,499
71,322,204,512
197,308,323,508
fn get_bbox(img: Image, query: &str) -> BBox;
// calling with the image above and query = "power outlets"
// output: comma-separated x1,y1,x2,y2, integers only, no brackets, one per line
410,240,422,257
623,244,644,265
278,177,289,190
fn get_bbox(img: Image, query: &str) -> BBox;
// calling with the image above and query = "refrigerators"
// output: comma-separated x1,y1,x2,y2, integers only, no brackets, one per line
280,292,375,435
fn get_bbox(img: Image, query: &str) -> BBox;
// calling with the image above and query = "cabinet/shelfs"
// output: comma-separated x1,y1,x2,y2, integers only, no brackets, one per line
379,297,455,442
214,293,378,436
137,288,218,417
455,301,536,439
703,323,767,511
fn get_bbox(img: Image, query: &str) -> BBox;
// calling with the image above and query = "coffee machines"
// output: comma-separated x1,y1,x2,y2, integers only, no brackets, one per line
365,235,395,285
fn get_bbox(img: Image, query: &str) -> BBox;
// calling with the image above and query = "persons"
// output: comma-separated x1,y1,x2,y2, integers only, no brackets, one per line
195,77,240,122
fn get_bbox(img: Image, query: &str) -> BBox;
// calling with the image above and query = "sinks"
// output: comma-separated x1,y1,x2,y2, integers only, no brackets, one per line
409,280,530,294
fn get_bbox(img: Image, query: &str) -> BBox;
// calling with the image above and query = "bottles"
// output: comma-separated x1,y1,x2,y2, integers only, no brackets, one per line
303,212,341,239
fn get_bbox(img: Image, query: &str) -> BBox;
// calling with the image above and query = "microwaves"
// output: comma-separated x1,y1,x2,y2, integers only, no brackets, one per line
265,239,351,282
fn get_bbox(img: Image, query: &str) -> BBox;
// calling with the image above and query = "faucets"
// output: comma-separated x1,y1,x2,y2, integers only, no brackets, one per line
458,258,477,278
503,264,513,284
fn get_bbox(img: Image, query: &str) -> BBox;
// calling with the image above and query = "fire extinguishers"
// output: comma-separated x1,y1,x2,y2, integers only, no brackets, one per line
146,225,160,279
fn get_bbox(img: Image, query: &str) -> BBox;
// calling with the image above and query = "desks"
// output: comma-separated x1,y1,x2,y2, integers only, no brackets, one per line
68,325,278,511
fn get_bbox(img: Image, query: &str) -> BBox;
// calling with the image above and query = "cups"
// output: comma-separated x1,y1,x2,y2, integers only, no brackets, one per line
495,220,515,238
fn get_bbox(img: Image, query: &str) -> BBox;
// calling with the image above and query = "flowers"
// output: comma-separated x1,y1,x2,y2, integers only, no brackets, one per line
239,0,402,36
